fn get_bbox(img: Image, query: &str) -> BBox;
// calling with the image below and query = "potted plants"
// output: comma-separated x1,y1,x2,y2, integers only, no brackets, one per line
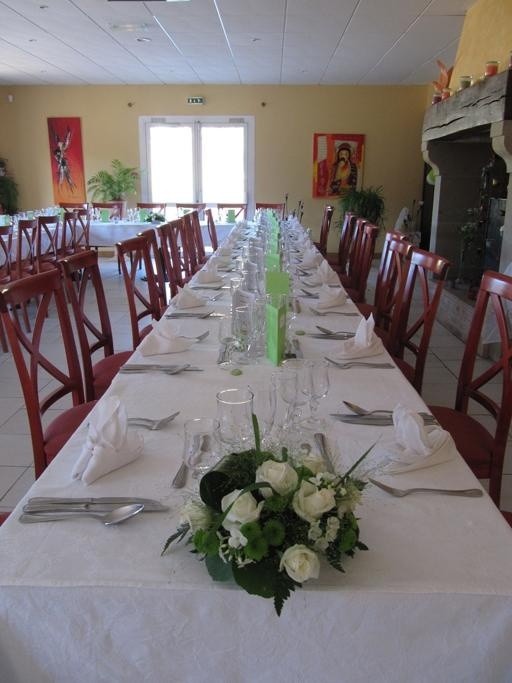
85,157,143,220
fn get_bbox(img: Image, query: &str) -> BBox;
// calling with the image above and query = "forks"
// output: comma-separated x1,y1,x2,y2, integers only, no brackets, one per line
368,477,483,499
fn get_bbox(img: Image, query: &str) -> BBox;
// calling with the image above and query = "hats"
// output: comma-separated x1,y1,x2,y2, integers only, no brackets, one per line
338,143,351,151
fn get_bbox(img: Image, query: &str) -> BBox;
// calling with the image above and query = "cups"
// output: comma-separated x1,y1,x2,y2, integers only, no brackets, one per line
430,60,500,105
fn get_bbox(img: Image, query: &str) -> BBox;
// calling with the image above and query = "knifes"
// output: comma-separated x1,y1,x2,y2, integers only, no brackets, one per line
23,504,171,513
328,413,435,421
28,495,160,505
340,419,439,426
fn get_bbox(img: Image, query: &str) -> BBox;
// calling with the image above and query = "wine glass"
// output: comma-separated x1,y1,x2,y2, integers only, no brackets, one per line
4,206,64,232
181,356,330,481
81,203,116,222
118,207,396,374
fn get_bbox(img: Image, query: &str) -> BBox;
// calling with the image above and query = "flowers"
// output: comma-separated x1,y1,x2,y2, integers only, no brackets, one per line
157,408,390,621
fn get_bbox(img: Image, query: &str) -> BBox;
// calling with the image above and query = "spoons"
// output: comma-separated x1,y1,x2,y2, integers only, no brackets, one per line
120,409,180,431
344,399,428,416
18,503,144,525
